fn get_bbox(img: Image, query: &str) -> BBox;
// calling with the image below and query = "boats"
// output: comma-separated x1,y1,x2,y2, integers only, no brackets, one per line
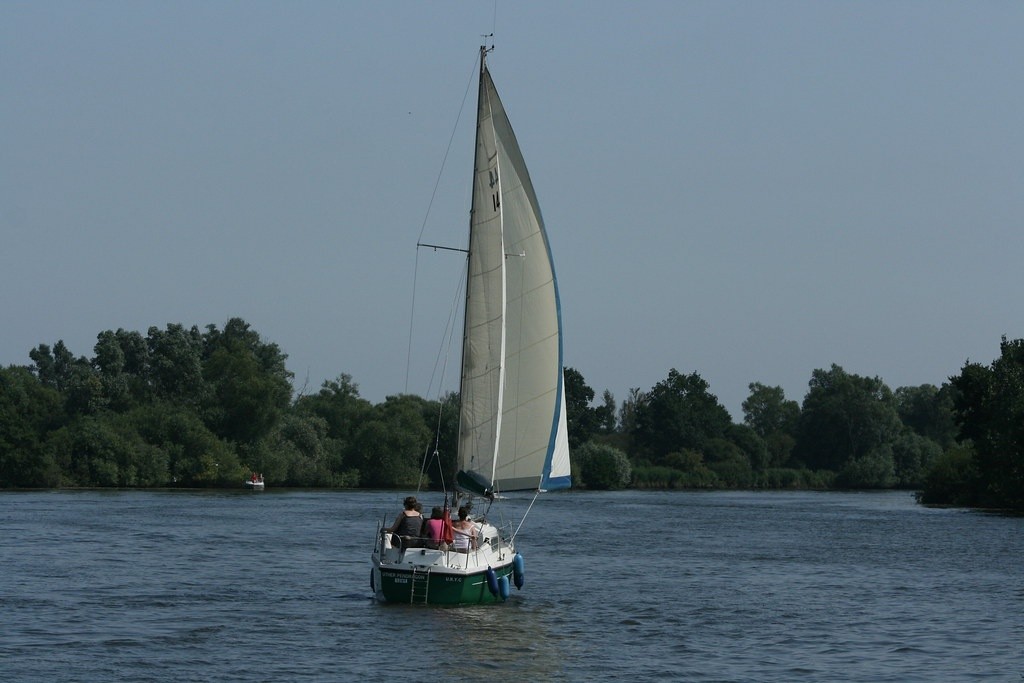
244,481,265,491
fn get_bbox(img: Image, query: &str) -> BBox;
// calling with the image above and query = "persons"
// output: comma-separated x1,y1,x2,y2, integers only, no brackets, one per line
386,496,476,553
251,472,264,483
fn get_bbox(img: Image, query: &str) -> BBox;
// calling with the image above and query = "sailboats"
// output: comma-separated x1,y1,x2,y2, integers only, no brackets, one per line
367,36,574,610
